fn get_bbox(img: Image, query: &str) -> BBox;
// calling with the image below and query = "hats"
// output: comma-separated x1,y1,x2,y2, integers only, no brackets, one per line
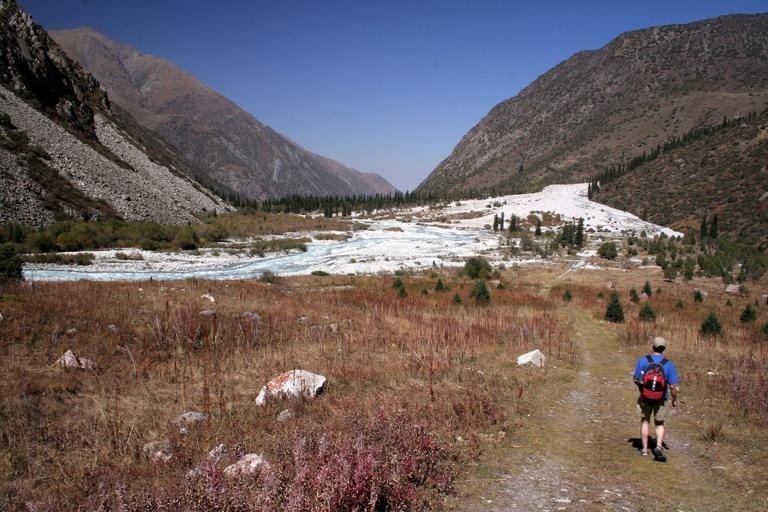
650,337,666,348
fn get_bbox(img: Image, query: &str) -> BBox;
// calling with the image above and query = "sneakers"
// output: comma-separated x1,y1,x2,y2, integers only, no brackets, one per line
653,448,666,462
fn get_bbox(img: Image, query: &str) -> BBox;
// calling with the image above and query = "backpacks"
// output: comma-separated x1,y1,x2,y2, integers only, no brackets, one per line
638,355,669,402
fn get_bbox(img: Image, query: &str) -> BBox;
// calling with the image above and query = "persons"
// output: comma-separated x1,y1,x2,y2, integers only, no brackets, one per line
633,337,680,462
